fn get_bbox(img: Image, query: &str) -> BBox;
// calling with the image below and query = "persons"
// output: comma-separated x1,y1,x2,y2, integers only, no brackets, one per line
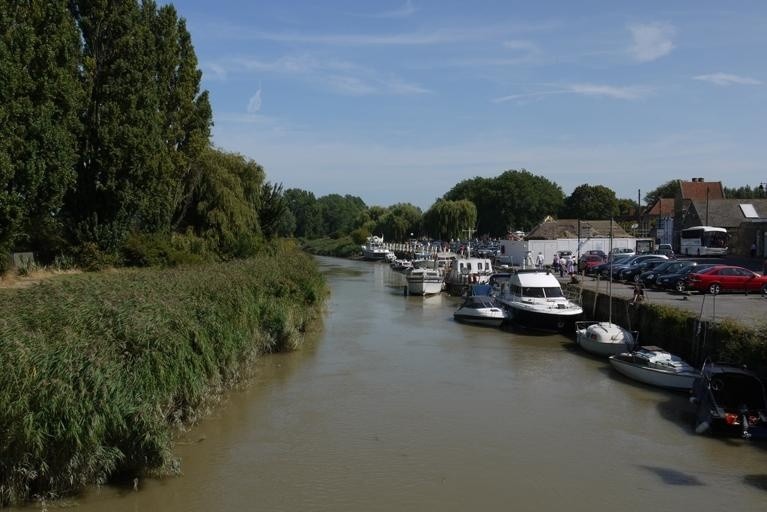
751,240,757,254
424,237,481,254
538,251,577,275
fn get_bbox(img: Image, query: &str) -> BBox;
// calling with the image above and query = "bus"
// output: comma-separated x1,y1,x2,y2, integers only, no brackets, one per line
678,225,730,258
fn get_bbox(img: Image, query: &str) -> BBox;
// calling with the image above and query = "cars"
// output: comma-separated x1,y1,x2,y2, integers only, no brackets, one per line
550,243,766,296
406,239,501,258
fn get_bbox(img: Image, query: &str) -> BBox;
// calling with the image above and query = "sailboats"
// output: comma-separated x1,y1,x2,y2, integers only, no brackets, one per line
575,218,639,359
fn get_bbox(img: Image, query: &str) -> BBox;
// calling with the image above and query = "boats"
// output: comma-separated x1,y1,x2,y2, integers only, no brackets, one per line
691,360,765,439
452,285,509,328
483,267,583,332
606,343,699,392
361,235,493,295
